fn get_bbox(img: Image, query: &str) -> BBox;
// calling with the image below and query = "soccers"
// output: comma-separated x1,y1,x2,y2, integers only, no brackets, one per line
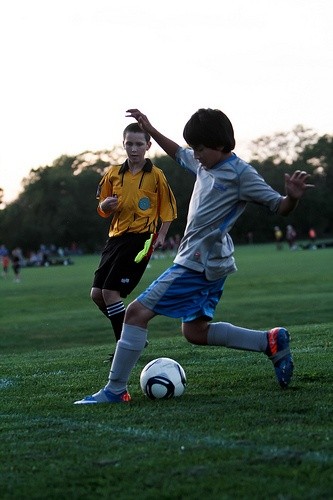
139,358,187,400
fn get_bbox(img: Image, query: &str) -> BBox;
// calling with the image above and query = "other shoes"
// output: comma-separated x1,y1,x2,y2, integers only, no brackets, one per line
104,340,149,365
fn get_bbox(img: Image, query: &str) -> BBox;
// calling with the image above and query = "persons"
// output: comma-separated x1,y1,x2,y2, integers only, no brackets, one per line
309,227,316,242
0,242,76,282
90,123,177,362
73,108,316,405
286,225,296,249
151,234,181,260
274,226,283,250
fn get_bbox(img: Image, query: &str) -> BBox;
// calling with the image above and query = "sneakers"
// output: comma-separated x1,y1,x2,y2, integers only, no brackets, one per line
73,387,132,405
264,327,294,388
